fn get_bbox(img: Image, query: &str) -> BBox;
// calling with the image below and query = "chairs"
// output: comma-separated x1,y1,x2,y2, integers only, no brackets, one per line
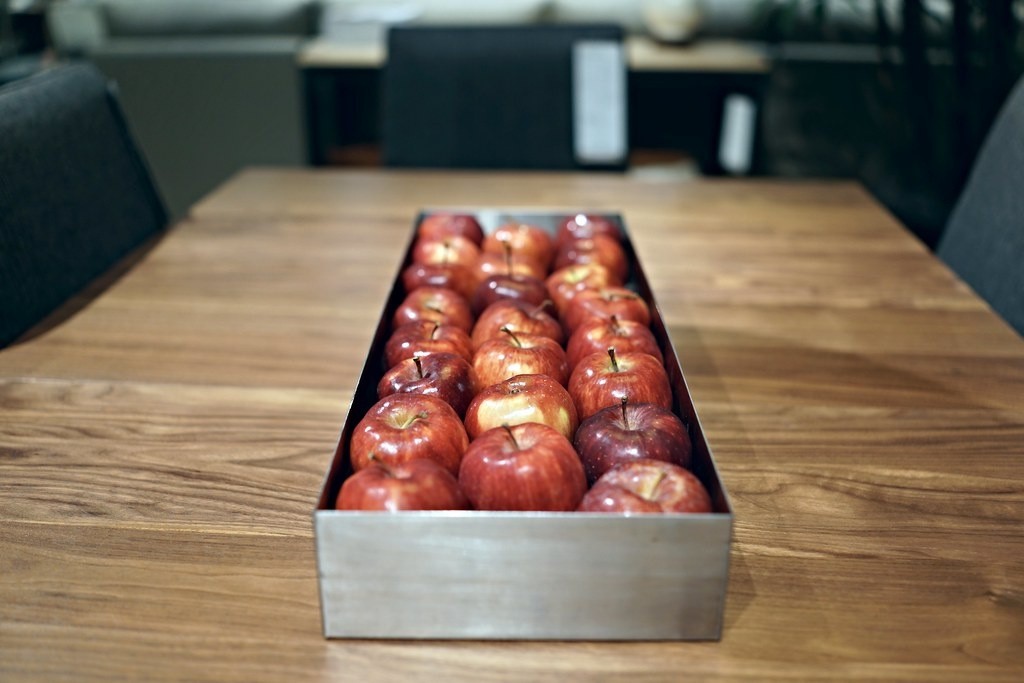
936,78,1024,336
384,19,636,174
0,51,173,349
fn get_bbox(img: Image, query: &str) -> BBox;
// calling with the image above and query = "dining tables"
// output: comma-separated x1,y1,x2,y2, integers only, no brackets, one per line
0,167,1024,683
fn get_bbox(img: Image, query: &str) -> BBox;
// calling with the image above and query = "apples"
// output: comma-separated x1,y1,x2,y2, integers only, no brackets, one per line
334,211,718,514
